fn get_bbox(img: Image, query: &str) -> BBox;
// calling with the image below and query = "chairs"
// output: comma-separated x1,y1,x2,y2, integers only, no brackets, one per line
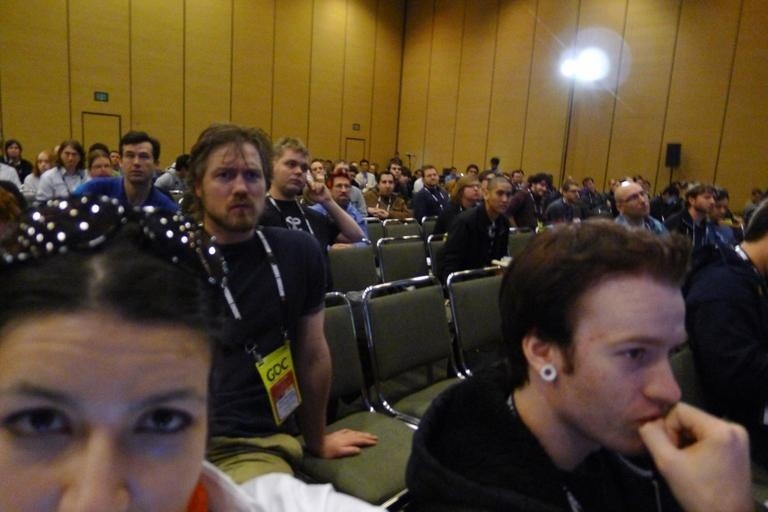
306,212,767,512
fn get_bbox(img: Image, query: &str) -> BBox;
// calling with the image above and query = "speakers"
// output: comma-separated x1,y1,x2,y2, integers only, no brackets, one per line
665,143,681,167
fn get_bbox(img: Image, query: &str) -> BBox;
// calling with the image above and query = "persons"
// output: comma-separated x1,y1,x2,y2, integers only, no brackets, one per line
678,199,768,487
405,219,768,512
1,129,190,213
178,125,378,485
306,155,767,296
260,137,366,245
2,196,389,512
